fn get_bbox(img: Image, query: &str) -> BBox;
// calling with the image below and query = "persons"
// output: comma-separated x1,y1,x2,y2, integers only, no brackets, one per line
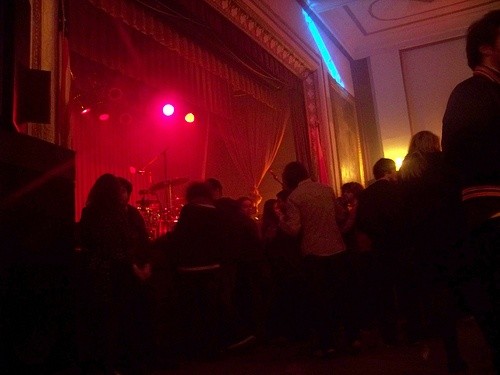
273,161,363,355
80,177,145,375
441,9,500,375
276,189,291,202
159,182,237,375
112,178,149,242
340,182,372,250
404,130,462,341
356,159,418,345
206,178,258,253
262,199,281,238
238,196,253,218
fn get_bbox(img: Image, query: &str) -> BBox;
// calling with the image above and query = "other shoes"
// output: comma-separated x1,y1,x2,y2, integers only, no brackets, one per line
228,334,256,351
313,349,336,358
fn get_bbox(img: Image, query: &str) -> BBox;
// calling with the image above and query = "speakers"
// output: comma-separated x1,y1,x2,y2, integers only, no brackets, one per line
19,69,51,125
